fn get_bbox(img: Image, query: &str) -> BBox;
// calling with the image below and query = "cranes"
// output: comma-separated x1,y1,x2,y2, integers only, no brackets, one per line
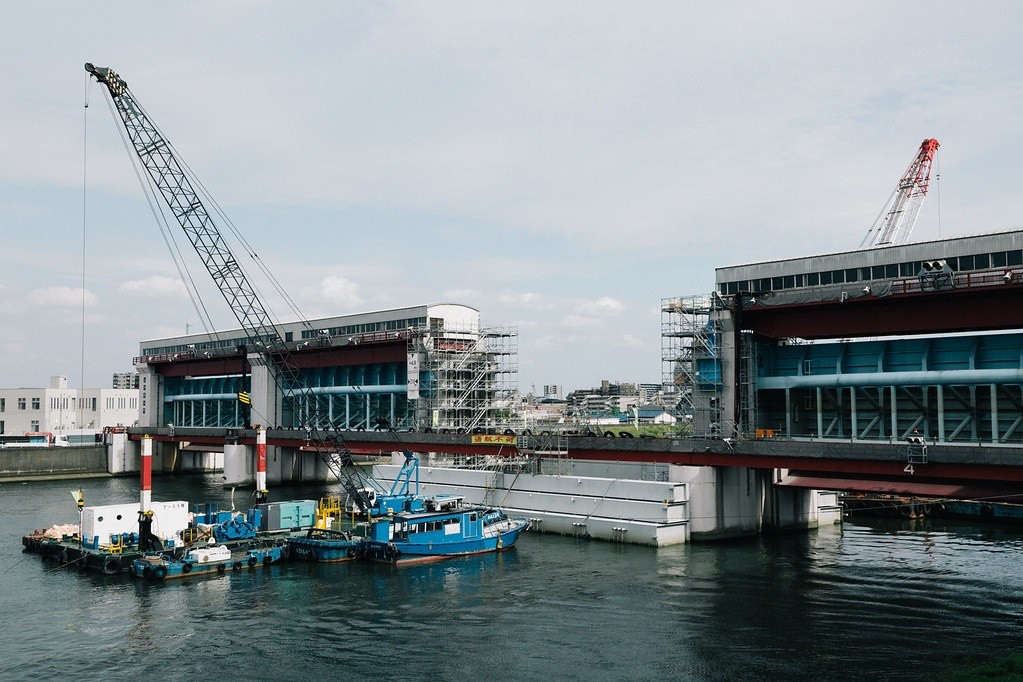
76,60,419,542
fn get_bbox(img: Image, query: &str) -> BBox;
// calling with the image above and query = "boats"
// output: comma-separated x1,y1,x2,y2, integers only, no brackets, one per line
379,493,529,562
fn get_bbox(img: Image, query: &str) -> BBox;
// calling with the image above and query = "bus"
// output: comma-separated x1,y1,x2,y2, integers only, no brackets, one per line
0,430,52,445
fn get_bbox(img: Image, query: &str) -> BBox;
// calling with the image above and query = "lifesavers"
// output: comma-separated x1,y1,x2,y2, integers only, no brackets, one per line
153,564,168,579
100,557,122,576
76,552,90,567
248,557,257,566
232,561,242,570
218,563,226,571
142,567,153,579
264,556,273,563
184,562,193,572
